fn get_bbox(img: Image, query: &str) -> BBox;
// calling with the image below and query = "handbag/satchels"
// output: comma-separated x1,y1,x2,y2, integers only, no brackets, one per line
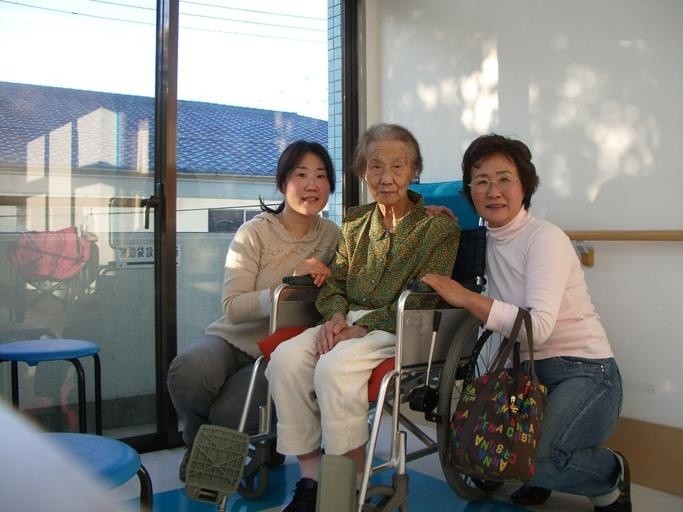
442,368,547,483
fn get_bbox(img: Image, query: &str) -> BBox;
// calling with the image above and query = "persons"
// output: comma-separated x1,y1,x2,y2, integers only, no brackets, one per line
265,123,461,511
165,139,342,502
420,133,631,512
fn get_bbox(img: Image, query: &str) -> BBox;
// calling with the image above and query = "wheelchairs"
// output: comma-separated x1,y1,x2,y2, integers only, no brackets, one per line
179,178,524,512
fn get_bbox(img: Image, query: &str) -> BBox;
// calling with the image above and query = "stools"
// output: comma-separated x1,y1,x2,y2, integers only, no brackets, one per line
42,431,152,512
1,339,102,434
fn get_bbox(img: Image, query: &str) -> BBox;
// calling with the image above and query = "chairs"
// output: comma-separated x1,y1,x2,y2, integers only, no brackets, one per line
8,228,90,327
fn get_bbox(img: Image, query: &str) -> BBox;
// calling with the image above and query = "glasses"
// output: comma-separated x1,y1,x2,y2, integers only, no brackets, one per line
466,175,520,194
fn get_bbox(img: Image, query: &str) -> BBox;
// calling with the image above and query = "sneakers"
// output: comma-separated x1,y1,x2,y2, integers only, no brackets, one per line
180,448,190,480
282,478,318,512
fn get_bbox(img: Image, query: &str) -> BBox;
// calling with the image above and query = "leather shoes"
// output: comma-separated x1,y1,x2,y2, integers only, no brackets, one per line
594,450,631,511
510,485,553,505
